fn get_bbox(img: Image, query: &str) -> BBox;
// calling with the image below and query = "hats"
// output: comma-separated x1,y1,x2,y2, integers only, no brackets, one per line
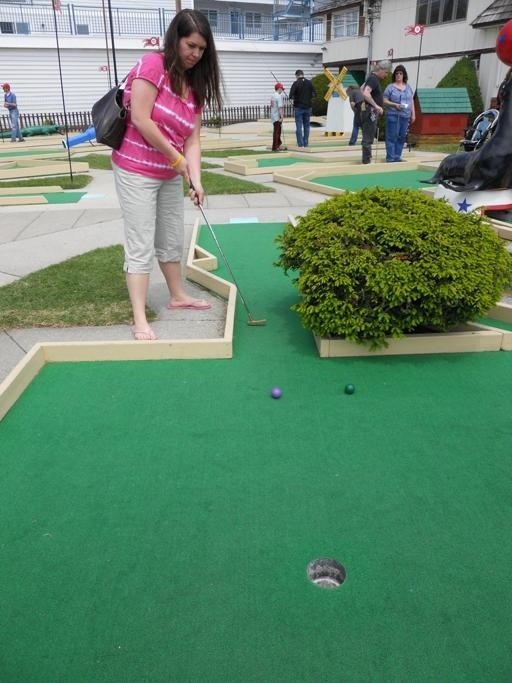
275,82,284,89
1,83,10,89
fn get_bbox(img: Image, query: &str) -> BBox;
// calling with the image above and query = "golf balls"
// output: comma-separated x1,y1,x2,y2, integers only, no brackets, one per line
271,386,283,399
344,383,356,395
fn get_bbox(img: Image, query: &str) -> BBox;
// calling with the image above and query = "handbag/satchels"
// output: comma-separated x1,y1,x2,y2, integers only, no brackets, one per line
91,84,129,150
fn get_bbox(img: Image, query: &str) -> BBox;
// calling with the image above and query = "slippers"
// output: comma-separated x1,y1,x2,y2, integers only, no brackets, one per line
130,319,157,340
167,298,212,309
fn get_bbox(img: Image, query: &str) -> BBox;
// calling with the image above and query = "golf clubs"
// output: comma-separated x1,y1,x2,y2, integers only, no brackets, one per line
280,121,287,151
375,111,386,164
189,178,268,327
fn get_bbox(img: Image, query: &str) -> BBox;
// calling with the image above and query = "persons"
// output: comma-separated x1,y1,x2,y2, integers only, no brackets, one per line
1,82,24,142
271,83,287,150
111,9,224,340
289,70,317,147
346,59,416,164
